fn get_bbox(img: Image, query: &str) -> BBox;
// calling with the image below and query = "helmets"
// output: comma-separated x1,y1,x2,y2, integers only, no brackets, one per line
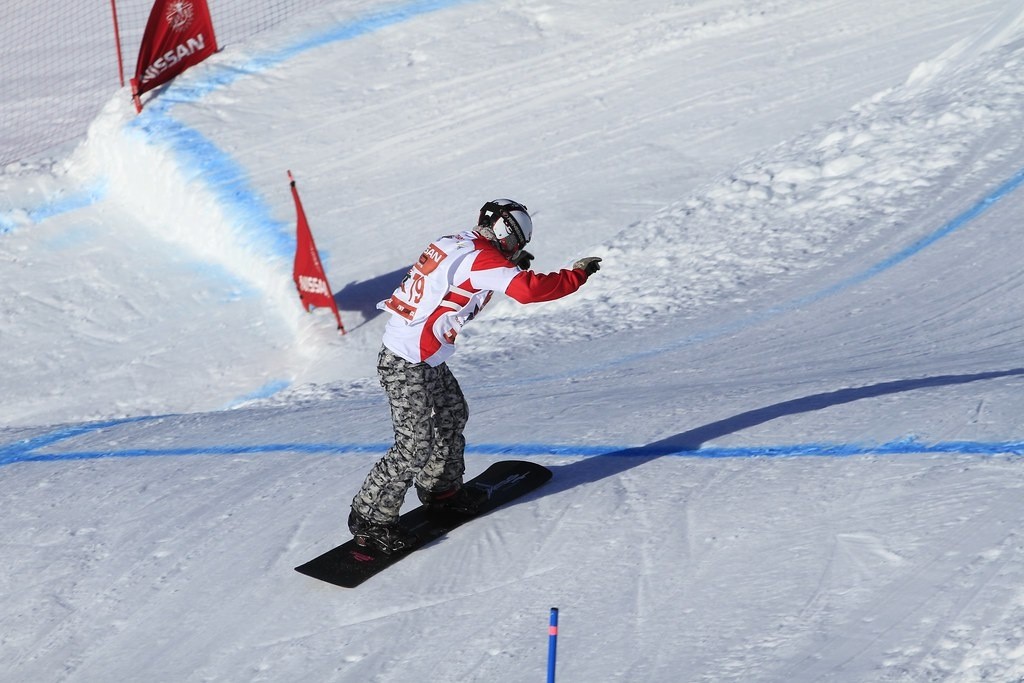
478,199,532,260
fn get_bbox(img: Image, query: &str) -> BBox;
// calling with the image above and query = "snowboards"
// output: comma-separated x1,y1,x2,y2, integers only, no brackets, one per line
293,459,554,588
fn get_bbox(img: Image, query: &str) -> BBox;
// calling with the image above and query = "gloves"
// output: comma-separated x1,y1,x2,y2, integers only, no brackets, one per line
573,257,602,277
514,250,535,270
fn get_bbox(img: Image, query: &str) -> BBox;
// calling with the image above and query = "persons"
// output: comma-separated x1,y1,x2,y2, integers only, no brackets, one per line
348,199,602,556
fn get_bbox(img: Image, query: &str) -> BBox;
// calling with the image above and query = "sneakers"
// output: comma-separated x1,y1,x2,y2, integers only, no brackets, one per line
415,483,481,510
348,505,420,557
